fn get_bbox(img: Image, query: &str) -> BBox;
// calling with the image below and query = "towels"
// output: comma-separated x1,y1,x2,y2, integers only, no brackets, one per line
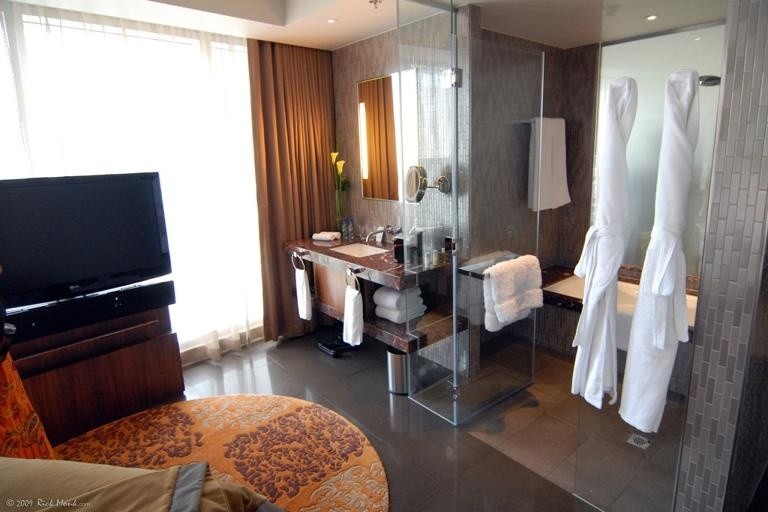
375,304,427,324
312,231,342,241
295,267,312,321
342,285,364,346
372,283,424,309
482,253,543,332
527,116,573,213
313,239,341,247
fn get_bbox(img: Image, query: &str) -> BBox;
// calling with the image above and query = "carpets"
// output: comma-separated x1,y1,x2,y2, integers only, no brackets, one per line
53,393,389,512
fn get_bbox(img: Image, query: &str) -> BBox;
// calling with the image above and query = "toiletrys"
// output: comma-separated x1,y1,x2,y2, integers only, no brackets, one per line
424,252,431,268
432,249,439,266
440,247,447,265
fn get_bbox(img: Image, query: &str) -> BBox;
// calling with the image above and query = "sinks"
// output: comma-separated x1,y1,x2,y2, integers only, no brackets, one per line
328,242,391,257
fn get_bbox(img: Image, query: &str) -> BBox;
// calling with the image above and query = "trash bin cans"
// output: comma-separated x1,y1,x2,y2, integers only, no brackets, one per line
386,345,418,396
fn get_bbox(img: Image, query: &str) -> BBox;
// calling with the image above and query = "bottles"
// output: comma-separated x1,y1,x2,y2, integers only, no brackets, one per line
341,218,354,241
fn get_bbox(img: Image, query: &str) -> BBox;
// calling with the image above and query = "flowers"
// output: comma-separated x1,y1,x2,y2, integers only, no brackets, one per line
330,152,345,175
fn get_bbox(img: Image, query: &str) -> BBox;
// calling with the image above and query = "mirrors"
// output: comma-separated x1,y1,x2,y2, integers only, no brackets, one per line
356,67,422,207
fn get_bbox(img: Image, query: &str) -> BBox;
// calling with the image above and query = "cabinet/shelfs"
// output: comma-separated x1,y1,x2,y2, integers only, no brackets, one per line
8,305,186,448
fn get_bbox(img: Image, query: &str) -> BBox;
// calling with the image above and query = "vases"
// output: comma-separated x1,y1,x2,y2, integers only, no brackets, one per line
335,174,349,232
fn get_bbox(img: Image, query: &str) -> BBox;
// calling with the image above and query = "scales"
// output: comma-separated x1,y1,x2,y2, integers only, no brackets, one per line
317,336,362,359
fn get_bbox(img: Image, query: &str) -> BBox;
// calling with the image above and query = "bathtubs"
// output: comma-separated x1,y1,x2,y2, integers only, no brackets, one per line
543,271,700,352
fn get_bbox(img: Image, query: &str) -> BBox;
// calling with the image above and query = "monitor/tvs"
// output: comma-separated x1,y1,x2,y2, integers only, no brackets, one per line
0,172,172,312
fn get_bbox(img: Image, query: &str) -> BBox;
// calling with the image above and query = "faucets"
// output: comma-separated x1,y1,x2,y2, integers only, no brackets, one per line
365,226,402,243
